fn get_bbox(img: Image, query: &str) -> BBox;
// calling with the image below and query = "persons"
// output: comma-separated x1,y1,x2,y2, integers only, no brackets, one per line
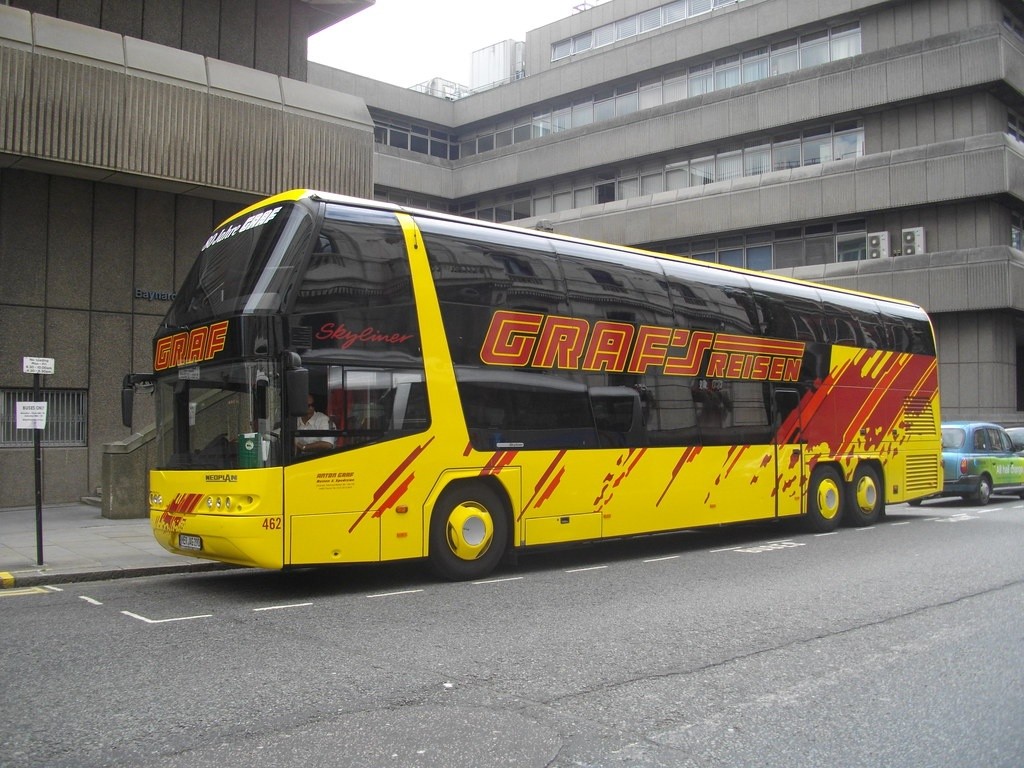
296,394,336,456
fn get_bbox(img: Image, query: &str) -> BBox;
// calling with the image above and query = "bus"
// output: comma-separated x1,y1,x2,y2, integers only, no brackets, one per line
120,188,946,583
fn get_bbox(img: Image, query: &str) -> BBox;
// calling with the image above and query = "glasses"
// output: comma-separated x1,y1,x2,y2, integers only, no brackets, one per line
308,403,315,408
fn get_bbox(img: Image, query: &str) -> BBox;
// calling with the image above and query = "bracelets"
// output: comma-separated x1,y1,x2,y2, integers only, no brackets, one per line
303,445,306,450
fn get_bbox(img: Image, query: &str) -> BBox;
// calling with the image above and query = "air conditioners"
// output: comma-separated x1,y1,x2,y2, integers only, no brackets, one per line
902,227,927,255
867,232,891,259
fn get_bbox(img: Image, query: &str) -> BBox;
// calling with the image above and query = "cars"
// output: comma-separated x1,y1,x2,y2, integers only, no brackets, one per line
993,427,1024,495
907,421,1024,507
